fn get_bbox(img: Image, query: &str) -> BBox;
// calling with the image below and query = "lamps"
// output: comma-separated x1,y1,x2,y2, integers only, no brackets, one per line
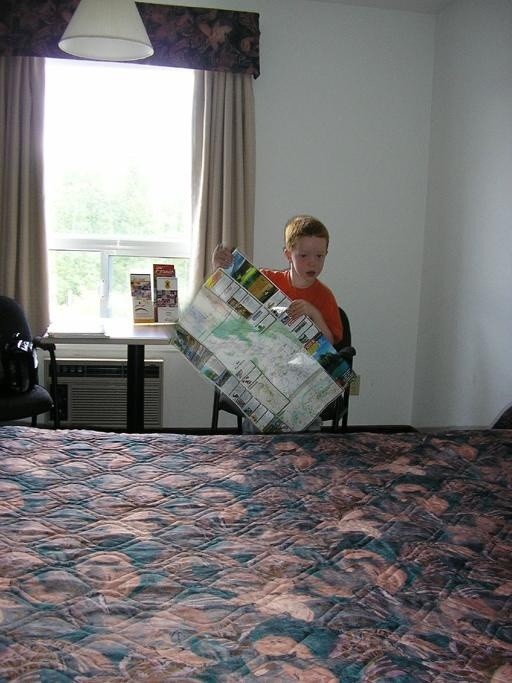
56,0,155,63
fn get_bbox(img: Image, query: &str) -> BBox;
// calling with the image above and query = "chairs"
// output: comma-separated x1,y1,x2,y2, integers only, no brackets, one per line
211,306,354,432
0,293,55,428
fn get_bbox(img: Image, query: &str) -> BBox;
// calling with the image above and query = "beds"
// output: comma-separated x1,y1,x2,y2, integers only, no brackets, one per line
0,423,512,683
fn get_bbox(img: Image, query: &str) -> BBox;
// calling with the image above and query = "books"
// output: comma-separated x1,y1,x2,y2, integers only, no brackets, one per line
48,321,109,339
129,263,180,324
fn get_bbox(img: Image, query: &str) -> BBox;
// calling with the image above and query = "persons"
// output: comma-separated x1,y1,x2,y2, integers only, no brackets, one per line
212,215,343,432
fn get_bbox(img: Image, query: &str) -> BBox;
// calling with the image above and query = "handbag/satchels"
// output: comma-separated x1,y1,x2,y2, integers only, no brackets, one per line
0,331,38,393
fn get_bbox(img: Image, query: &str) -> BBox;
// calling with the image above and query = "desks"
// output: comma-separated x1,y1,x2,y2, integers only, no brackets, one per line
40,323,171,432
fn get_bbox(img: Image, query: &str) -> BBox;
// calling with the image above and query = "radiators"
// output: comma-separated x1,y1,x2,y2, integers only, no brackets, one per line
42,357,165,428
